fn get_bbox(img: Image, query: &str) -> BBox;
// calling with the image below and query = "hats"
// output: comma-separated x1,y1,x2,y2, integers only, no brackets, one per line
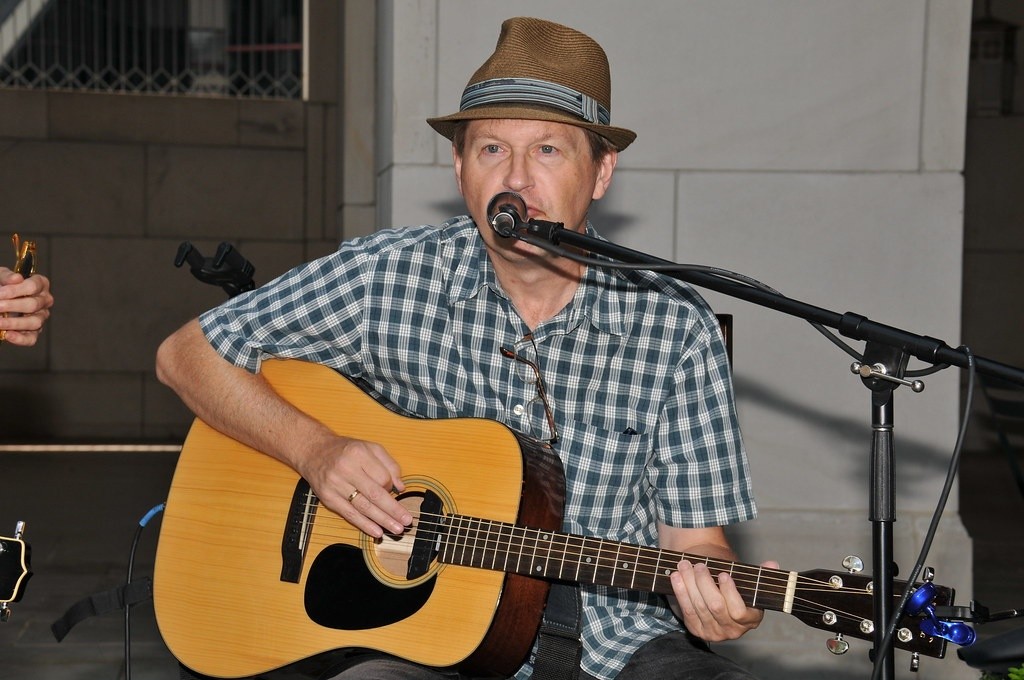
426,15,637,153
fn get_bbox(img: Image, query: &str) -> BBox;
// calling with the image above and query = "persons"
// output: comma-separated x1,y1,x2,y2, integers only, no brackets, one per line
0,265,55,349
155,19,768,680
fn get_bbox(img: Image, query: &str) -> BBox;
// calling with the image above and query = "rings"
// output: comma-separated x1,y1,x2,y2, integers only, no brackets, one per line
348,490,359,503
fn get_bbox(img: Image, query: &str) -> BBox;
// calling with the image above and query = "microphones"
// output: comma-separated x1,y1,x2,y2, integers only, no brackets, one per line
486,190,528,243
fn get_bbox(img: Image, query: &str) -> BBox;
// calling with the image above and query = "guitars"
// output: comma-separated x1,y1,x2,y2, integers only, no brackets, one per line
151,358,1019,678
